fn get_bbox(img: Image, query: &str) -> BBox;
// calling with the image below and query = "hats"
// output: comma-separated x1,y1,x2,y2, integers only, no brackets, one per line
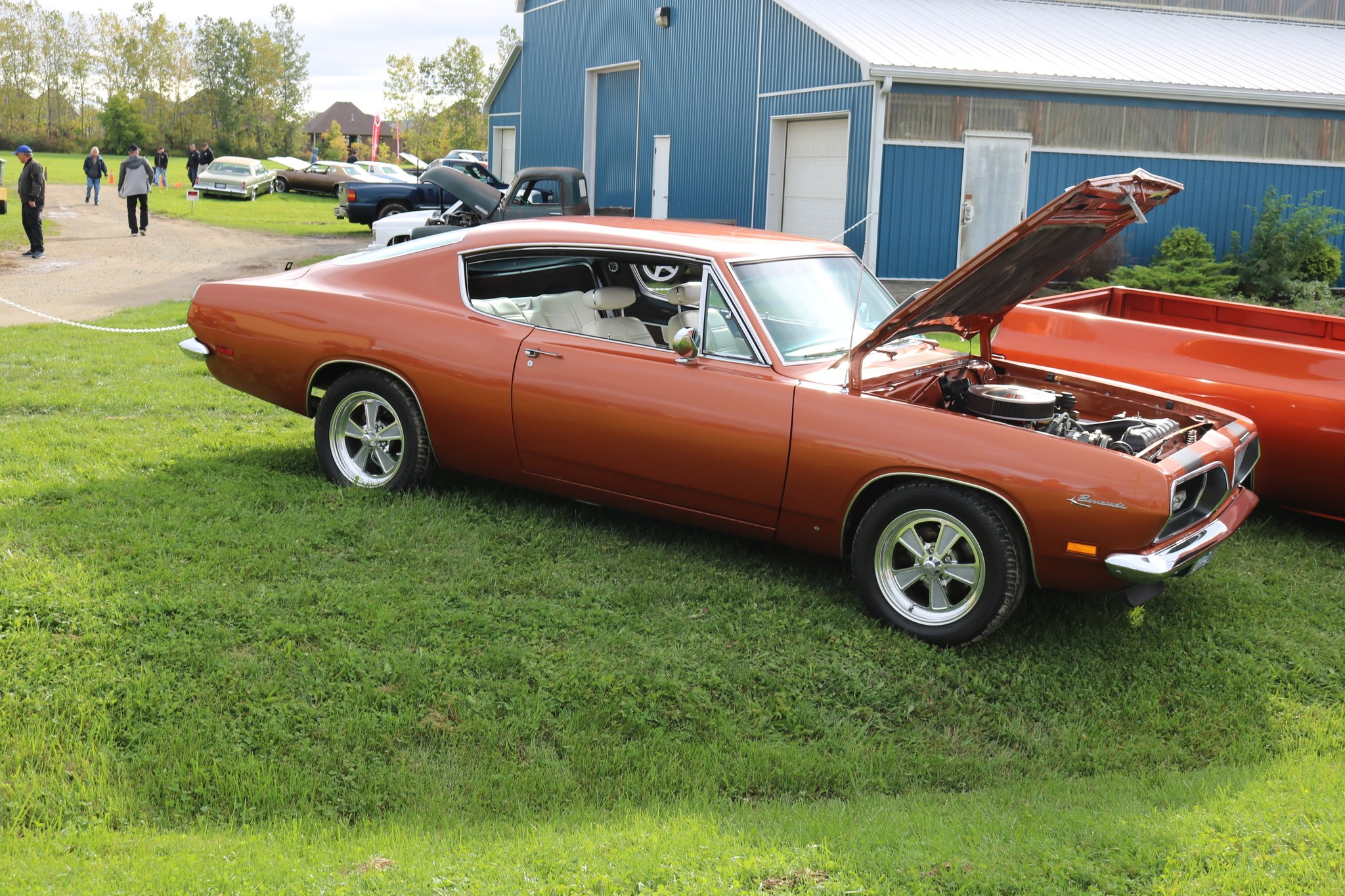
128,144,140,151
12,145,33,155
156,146,162,151
202,143,208,146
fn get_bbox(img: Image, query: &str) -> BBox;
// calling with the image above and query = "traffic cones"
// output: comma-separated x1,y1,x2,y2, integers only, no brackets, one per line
176,181,182,188
160,176,163,187
110,169,114,184
44,166,48,180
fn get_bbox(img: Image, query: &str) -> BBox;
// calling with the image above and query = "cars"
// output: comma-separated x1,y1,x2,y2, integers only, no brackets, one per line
267,149,488,198
193,156,278,202
178,169,1262,654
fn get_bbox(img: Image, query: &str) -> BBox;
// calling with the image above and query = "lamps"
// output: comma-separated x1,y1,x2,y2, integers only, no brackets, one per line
654,6,670,29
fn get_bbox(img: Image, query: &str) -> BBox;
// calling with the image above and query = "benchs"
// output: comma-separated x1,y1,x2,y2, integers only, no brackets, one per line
471,290,601,334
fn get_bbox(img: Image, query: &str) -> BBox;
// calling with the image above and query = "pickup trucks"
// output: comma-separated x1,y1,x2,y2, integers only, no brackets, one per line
412,166,737,285
989,286,1345,525
334,163,557,232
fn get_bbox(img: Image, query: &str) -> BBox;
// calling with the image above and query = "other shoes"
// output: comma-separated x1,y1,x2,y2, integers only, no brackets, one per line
95,202,98,205
22,250,35,257
32,249,45,259
85,197,89,202
131,232,137,236
140,229,147,236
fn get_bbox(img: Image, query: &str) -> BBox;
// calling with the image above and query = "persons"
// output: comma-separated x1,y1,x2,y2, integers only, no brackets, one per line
154,146,168,187
83,146,107,206
118,143,154,237
347,150,358,163
196,142,214,177
309,148,319,164
186,143,200,187
12,145,46,259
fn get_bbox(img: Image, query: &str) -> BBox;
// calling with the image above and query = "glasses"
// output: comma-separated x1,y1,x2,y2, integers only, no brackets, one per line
202,146,206,148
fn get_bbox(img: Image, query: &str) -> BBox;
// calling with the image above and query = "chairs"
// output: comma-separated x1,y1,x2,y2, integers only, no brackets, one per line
667,282,741,356
581,286,657,348
